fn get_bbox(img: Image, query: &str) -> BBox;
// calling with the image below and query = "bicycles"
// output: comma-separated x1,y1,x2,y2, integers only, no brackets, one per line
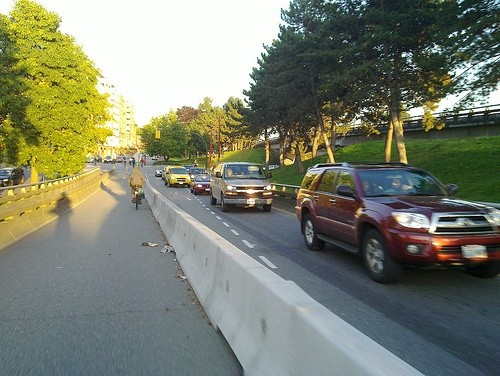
130,184,145,211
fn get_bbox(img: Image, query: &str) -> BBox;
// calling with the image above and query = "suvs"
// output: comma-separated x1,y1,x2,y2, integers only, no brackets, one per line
294,160,500,286
0,167,25,187
210,162,273,212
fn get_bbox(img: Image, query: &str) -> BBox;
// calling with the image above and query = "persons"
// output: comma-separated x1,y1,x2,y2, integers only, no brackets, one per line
94,155,96,165
132,157,135,167
128,166,146,203
385,173,416,195
193,159,198,167
123,155,127,165
361,180,374,194
226,168,234,176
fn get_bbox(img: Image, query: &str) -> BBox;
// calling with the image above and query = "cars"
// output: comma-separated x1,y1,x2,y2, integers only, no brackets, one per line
85,155,127,163
154,164,211,195
